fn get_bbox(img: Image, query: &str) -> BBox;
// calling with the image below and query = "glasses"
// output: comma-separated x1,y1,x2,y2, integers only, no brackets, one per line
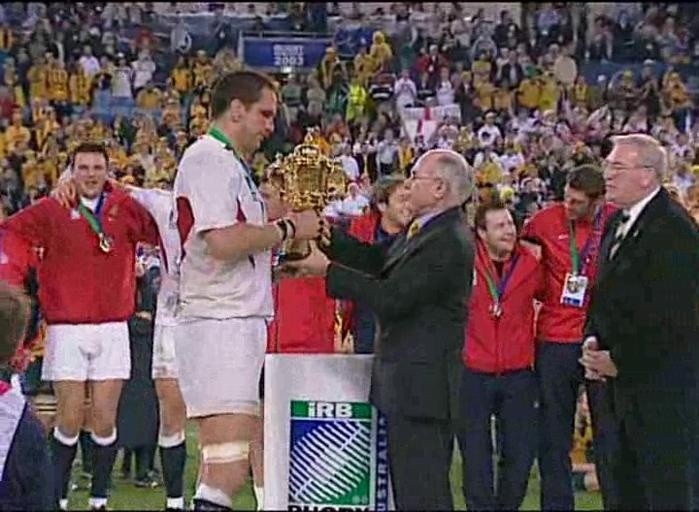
410,171,435,181
602,159,652,174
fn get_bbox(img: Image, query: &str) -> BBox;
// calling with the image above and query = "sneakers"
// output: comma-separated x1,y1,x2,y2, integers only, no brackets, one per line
52,466,264,511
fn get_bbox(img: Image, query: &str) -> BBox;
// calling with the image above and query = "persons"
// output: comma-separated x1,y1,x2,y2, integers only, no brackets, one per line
174,71,318,511
1,0,699,150
2,134,699,511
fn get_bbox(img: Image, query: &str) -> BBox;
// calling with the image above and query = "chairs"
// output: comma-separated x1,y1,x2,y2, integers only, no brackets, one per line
581,62,665,89
91,88,163,125
